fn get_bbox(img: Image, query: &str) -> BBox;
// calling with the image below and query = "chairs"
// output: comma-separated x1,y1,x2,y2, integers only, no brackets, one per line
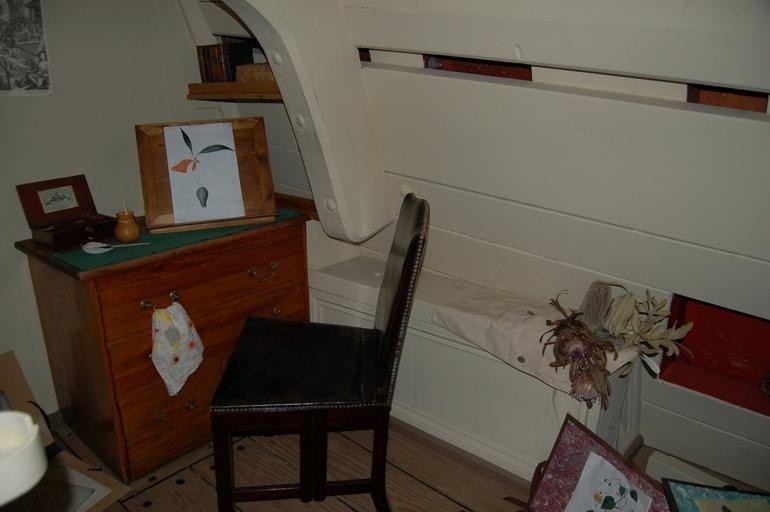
206,191,430,511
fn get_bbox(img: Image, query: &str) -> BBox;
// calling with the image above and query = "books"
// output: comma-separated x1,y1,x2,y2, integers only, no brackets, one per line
196,37,268,82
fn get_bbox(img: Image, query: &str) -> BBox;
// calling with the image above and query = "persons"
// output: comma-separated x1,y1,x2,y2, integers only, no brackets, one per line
29,50,48,89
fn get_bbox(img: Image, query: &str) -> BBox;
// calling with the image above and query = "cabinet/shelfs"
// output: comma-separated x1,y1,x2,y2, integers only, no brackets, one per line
15,202,313,485
311,256,643,485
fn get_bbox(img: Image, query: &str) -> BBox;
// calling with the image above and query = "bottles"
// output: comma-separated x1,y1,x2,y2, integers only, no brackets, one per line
115,210,140,243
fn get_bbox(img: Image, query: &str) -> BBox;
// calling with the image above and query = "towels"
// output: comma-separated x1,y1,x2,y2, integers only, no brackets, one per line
149,302,205,397
